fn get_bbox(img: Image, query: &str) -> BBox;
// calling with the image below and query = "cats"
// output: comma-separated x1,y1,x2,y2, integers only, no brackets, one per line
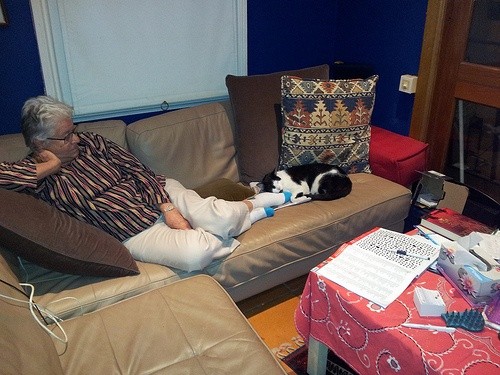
256,162,352,203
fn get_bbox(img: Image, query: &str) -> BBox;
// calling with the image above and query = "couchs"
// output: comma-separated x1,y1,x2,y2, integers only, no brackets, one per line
0,97,412,375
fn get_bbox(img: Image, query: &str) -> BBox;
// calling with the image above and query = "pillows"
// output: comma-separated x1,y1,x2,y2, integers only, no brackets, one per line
280,73,378,175
225,65,329,186
0,188,141,279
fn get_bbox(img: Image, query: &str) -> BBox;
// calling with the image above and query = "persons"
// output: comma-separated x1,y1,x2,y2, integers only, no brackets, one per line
0,96,293,272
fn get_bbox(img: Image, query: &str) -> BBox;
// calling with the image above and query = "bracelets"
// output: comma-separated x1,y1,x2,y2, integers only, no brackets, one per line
162,204,177,214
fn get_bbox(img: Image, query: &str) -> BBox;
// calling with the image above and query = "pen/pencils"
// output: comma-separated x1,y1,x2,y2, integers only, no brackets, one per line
387,249,430,260
401,323,457,333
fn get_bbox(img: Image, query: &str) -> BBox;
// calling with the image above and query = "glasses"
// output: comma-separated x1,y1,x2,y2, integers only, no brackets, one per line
46,123,79,143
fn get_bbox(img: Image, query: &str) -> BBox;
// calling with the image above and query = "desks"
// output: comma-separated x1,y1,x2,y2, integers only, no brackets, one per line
307,208,500,375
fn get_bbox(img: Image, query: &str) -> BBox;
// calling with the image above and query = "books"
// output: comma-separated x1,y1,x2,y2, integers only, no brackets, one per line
311,226,443,308
